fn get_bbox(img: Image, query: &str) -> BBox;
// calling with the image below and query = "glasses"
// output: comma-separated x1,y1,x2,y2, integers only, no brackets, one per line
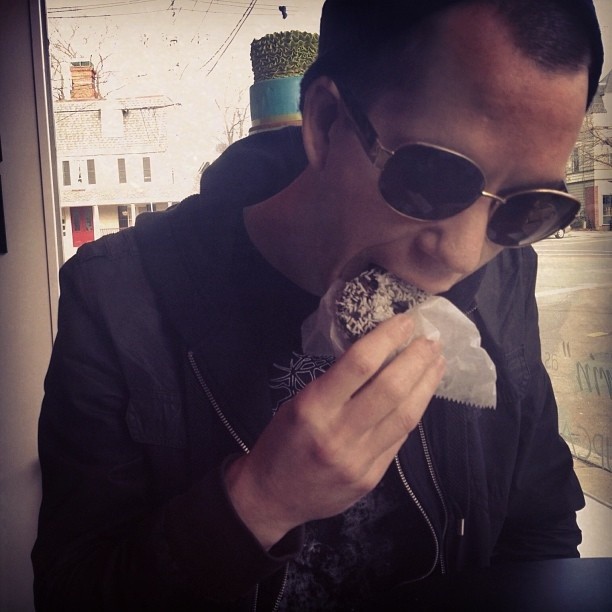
332,76,581,249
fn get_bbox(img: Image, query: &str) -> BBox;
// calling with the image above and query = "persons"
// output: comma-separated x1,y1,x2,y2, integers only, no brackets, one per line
31,0,603,612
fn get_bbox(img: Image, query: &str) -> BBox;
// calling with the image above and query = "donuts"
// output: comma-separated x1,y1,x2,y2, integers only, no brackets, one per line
334,265,432,344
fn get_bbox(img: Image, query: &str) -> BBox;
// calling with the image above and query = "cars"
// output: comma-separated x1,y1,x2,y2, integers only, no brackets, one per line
552,224,571,238
525,202,556,224
